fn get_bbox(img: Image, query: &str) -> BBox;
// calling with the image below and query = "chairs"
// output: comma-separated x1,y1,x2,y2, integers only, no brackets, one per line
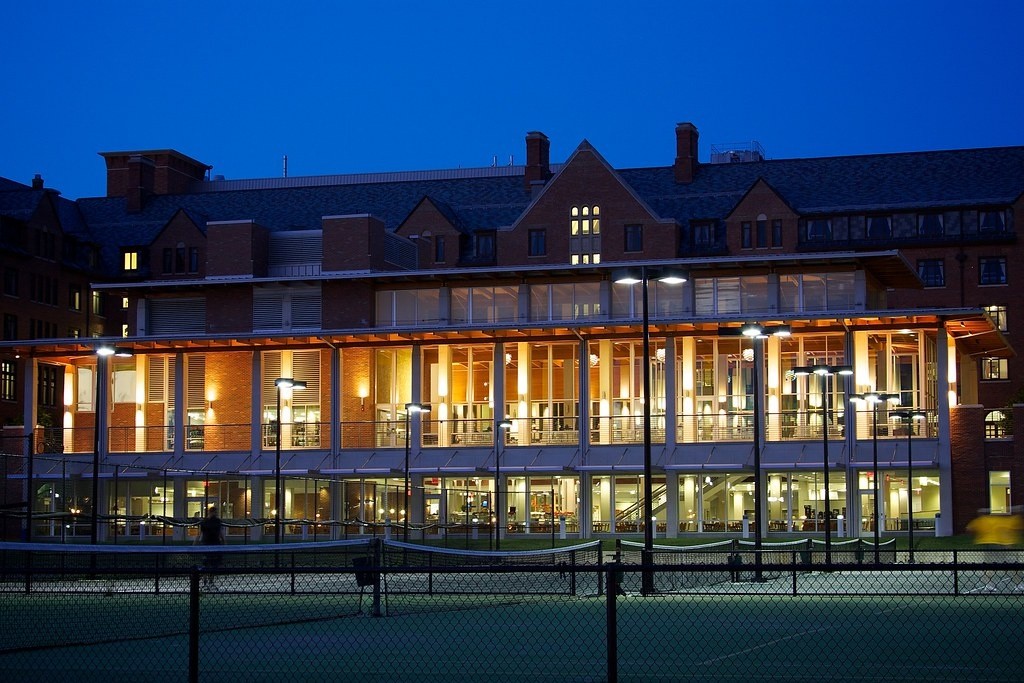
506,519,903,535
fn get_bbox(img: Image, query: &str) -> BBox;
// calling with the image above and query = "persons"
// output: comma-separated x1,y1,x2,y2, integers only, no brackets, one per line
193,508,226,584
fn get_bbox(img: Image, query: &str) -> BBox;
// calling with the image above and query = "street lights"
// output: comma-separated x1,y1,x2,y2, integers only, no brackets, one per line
844,390,901,566
399,402,431,565
792,364,857,572
740,324,793,581
609,263,693,601
495,420,513,566
271,376,308,568
87,344,134,580
887,411,930,565
